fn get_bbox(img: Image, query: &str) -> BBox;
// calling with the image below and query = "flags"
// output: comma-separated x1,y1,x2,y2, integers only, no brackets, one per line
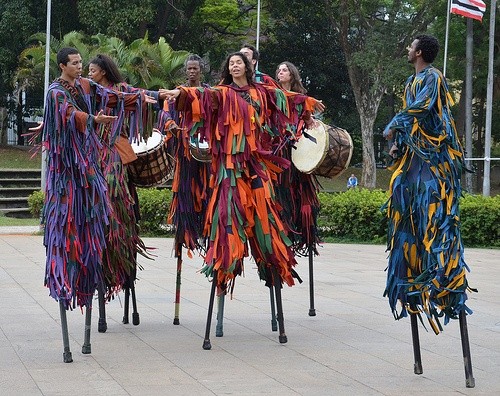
450,0,487,23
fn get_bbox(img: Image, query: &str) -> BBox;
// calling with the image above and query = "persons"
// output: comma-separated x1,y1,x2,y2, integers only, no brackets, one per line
44,48,161,294
156,43,326,283
383,34,473,297
89,53,173,269
346,174,358,190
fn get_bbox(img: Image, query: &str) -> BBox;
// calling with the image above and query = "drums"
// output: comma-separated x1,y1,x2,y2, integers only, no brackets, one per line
189,135,214,163
128,126,174,189
290,118,355,179
